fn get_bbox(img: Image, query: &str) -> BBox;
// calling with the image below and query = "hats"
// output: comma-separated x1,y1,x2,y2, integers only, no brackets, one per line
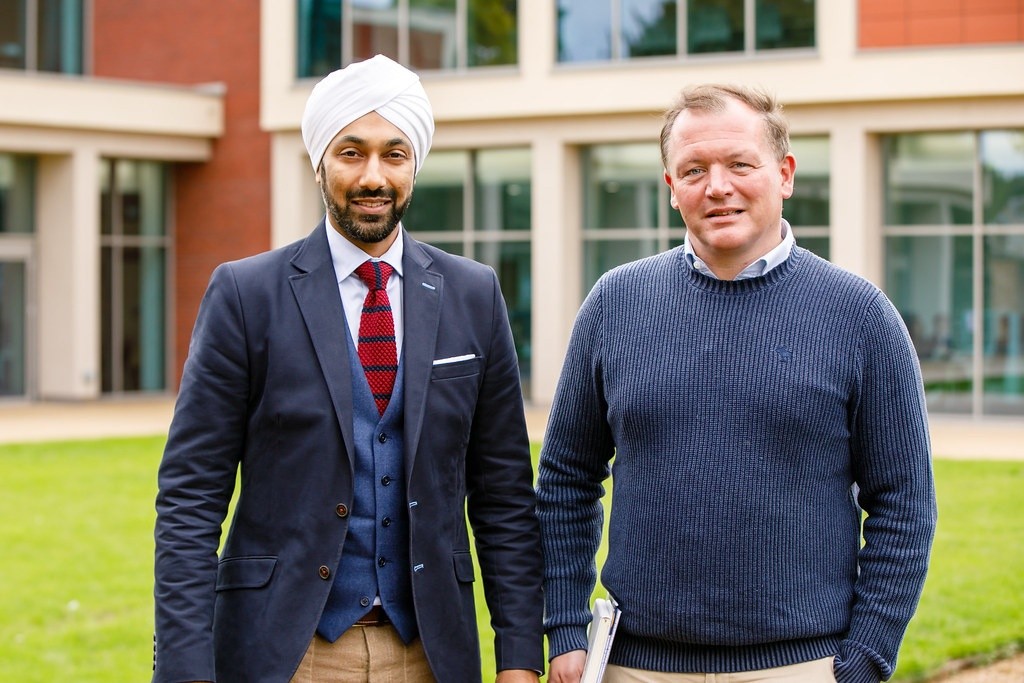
301,54,434,177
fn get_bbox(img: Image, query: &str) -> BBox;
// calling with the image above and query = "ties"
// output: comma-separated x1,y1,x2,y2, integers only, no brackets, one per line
354,260,397,419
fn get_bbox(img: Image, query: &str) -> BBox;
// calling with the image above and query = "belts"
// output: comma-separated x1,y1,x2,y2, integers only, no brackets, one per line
352,605,391,627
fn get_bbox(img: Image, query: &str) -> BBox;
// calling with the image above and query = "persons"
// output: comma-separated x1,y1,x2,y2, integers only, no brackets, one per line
535,78,938,683
150,52,552,683
